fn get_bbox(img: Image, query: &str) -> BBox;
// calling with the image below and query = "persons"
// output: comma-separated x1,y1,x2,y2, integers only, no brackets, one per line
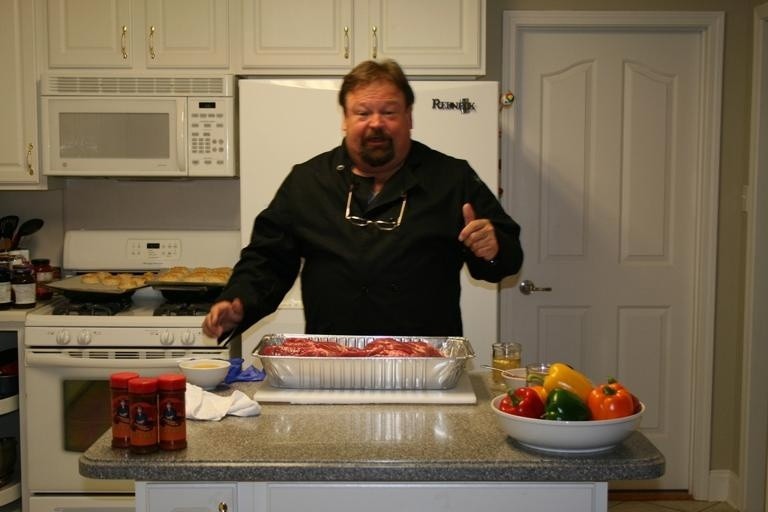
203,58,523,338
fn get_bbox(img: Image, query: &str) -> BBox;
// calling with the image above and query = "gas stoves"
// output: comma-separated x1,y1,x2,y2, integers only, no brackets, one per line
23,294,241,348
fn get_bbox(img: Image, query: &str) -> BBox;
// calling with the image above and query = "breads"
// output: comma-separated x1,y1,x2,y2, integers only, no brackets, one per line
82,266,233,290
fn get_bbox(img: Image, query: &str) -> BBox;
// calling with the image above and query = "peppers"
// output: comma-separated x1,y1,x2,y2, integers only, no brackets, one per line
501,363,640,421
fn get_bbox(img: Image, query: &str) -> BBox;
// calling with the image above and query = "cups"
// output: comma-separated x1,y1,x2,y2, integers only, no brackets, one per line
0,248,30,273
524,364,551,389
491,342,521,386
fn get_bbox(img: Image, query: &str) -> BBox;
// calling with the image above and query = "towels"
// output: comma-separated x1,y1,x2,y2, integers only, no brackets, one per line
184,383,261,422
225,358,266,382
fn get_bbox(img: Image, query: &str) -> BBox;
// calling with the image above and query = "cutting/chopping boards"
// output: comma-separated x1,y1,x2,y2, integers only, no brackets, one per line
254,371,477,406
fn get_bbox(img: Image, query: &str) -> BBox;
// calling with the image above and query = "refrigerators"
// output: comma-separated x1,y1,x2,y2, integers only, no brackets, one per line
236,75,499,367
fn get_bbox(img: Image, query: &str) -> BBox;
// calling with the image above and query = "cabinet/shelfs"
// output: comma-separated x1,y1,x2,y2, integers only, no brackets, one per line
46,1,237,77
237,1,489,81
0,1,67,193
131,480,236,510
237,481,609,511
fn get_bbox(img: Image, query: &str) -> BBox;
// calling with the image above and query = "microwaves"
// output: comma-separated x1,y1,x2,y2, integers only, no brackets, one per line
37,71,236,180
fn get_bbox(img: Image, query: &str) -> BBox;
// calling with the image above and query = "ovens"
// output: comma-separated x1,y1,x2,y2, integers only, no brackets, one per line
21,349,239,510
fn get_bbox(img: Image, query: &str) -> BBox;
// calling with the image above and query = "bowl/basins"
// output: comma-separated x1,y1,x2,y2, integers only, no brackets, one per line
178,360,231,390
491,392,645,455
500,367,524,390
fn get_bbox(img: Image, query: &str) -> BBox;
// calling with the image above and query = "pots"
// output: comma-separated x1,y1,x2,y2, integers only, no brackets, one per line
0,346,19,489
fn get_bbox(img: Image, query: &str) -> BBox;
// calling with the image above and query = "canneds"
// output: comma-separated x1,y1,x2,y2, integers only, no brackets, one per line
0,258,54,309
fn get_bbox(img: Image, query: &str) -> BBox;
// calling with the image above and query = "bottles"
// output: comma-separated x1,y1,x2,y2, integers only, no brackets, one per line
108,372,187,453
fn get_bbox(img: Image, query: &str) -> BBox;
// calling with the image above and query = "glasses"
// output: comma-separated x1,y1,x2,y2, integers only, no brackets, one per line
345,191,408,234
